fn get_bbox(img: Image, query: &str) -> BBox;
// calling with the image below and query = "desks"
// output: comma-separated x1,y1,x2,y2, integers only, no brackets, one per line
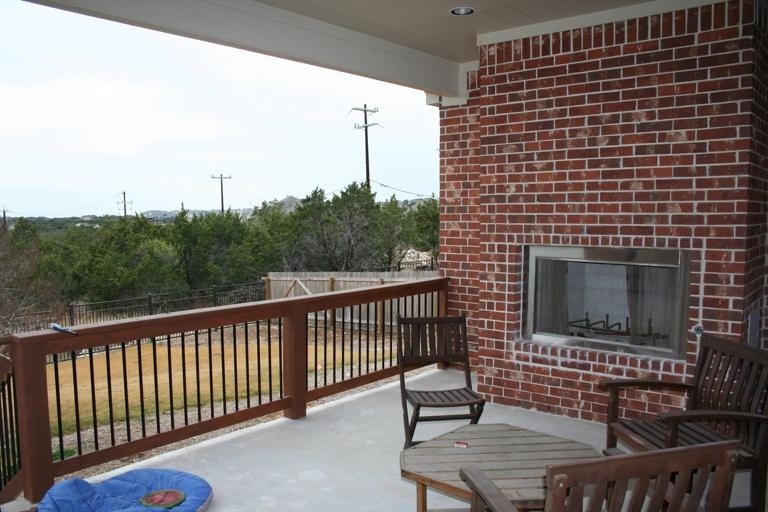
399,423,604,512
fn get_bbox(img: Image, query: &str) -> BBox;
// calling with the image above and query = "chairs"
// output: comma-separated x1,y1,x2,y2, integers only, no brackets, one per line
395,312,487,448
